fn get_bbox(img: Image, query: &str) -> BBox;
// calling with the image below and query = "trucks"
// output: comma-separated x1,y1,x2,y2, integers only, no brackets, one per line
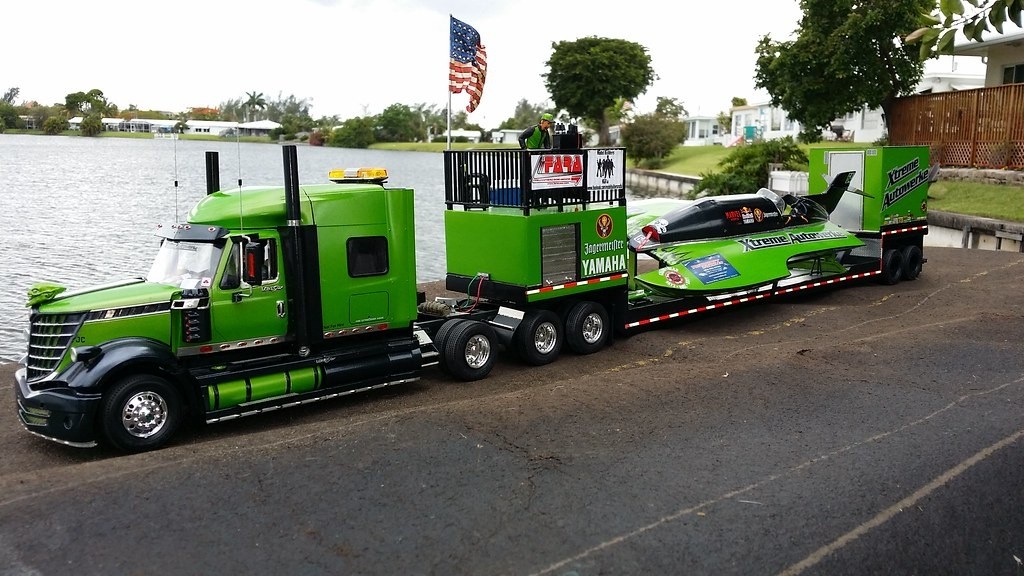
15,125,931,455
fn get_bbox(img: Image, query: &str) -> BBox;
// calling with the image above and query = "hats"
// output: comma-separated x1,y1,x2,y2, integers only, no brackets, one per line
541,112,556,123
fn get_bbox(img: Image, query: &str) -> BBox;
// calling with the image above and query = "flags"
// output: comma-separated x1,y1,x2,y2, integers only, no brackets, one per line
449,14,487,113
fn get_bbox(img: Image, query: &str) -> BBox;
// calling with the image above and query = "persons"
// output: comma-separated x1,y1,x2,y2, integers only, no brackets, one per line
518,113,556,150
597,155,615,178
